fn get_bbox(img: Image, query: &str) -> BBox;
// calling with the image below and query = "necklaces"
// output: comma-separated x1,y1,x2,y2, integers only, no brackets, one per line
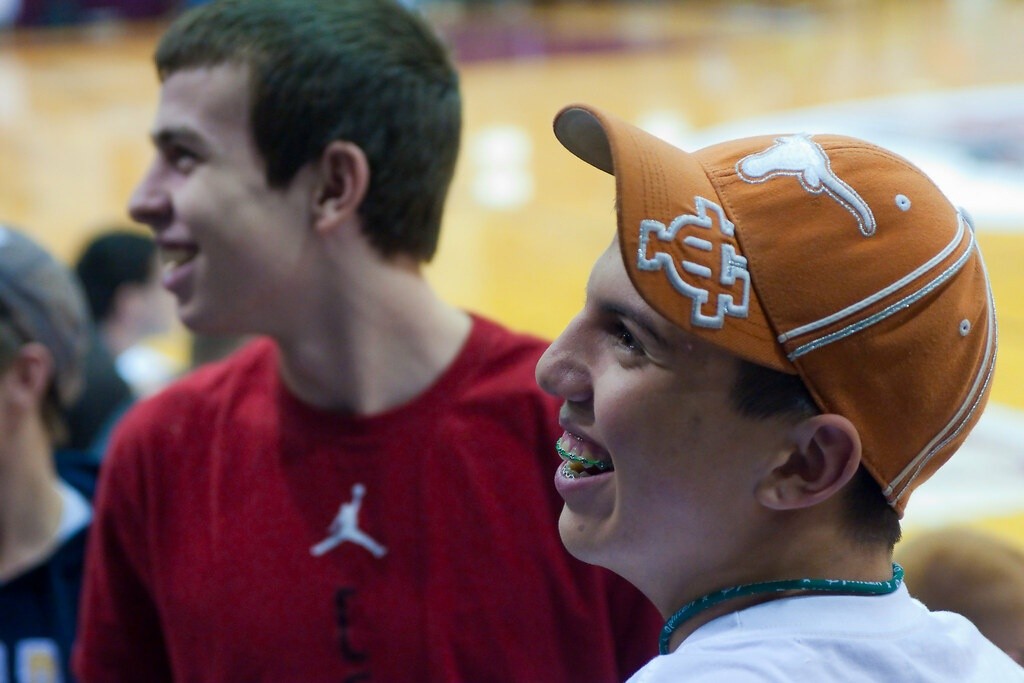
659,557,907,655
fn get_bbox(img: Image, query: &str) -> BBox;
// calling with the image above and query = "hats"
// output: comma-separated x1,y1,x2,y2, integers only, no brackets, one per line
550,103,998,521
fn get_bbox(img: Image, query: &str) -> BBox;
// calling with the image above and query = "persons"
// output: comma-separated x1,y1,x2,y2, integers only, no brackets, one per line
531,100,1024,683
58,231,191,456
0,226,107,683
68,2,667,683
